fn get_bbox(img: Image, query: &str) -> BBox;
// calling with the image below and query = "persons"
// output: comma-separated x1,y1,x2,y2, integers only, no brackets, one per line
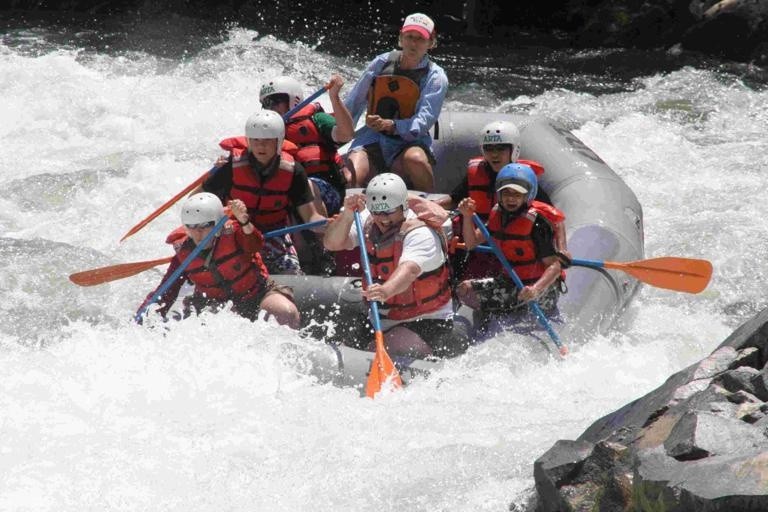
455,164,561,311
322,172,455,358
215,75,354,218
187,112,337,276
432,120,572,264
339,12,450,197
136,193,302,330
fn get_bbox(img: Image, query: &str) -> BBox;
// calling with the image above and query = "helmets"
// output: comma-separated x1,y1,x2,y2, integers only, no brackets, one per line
244,76,305,152
181,192,224,237
364,172,409,220
481,121,539,206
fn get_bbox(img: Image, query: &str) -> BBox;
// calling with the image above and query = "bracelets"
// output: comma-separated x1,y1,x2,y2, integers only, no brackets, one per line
236,219,250,226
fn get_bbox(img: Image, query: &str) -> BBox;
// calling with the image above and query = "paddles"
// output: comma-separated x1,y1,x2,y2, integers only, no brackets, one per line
449,237,714,293
69,215,337,288
120,82,331,240
350,205,401,397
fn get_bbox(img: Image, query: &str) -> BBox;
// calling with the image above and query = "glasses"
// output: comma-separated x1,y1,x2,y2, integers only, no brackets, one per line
185,222,213,231
482,144,511,152
370,209,400,216
262,94,289,109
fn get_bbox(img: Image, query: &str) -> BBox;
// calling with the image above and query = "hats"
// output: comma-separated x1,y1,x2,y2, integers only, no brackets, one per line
400,12,434,40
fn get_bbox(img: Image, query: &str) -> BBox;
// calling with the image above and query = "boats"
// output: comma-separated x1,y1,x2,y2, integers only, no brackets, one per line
238,109,645,398
